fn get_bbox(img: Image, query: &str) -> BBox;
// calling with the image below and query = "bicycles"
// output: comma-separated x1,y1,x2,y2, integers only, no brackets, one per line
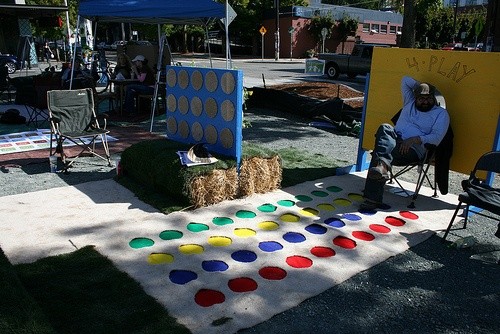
83,62,110,94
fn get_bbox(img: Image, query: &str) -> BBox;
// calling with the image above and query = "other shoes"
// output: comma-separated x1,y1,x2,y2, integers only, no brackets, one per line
361,201,376,210
128,112,136,118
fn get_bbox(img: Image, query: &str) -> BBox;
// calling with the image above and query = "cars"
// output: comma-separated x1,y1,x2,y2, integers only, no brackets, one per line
442,42,484,51
33,39,128,63
0,52,23,74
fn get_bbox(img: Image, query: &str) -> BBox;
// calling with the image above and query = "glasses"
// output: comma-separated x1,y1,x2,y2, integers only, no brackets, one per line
416,97,433,102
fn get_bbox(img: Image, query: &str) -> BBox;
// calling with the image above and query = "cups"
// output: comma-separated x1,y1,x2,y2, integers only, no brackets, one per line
131,71,135,79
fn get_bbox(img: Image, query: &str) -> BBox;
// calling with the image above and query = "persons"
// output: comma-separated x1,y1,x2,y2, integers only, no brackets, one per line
359,77,450,213
44,43,155,119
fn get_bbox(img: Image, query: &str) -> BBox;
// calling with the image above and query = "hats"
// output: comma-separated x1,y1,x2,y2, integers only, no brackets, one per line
415,83,435,95
131,55,145,62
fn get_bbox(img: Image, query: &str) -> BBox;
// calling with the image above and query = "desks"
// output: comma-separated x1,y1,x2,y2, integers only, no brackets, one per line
109,78,139,120
15,75,60,128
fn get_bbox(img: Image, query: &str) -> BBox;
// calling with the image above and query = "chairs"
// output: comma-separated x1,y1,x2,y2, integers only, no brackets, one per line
137,81,154,120
381,108,453,209
441,151,500,243
47,87,114,175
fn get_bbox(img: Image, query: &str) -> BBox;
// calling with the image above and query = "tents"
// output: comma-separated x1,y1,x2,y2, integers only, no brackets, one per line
69,0,238,133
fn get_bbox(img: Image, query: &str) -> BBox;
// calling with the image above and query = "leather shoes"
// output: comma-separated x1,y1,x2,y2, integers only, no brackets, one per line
369,161,390,180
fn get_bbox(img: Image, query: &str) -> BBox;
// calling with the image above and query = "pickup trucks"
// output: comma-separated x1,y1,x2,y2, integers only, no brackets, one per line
319,43,391,80
